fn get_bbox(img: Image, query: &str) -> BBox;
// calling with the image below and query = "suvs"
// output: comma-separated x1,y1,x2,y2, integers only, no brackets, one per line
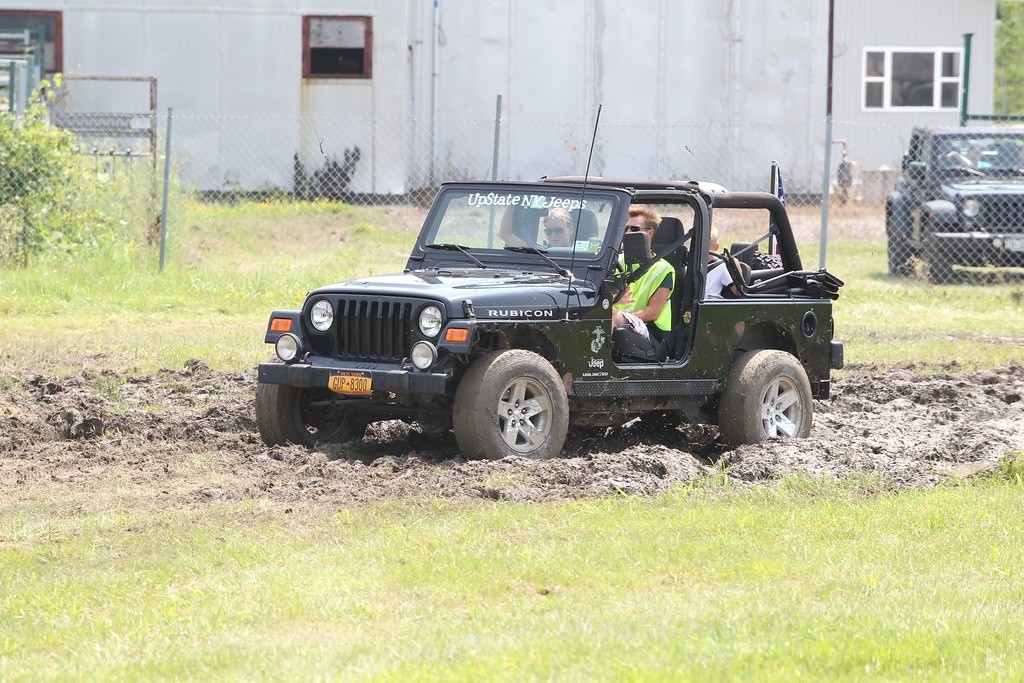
885,122,1024,287
254,173,844,465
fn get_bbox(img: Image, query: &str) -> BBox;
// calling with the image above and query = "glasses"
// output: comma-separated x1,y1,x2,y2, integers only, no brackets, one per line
624,226,652,232
543,227,565,236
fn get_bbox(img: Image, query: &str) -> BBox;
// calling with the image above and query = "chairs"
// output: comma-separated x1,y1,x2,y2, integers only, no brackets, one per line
993,141,1019,168
614,217,686,361
566,208,599,242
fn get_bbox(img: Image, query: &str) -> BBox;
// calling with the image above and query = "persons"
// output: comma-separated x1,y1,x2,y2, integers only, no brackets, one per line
611,208,676,362
948,139,993,170
685,227,741,299
498,194,602,250
